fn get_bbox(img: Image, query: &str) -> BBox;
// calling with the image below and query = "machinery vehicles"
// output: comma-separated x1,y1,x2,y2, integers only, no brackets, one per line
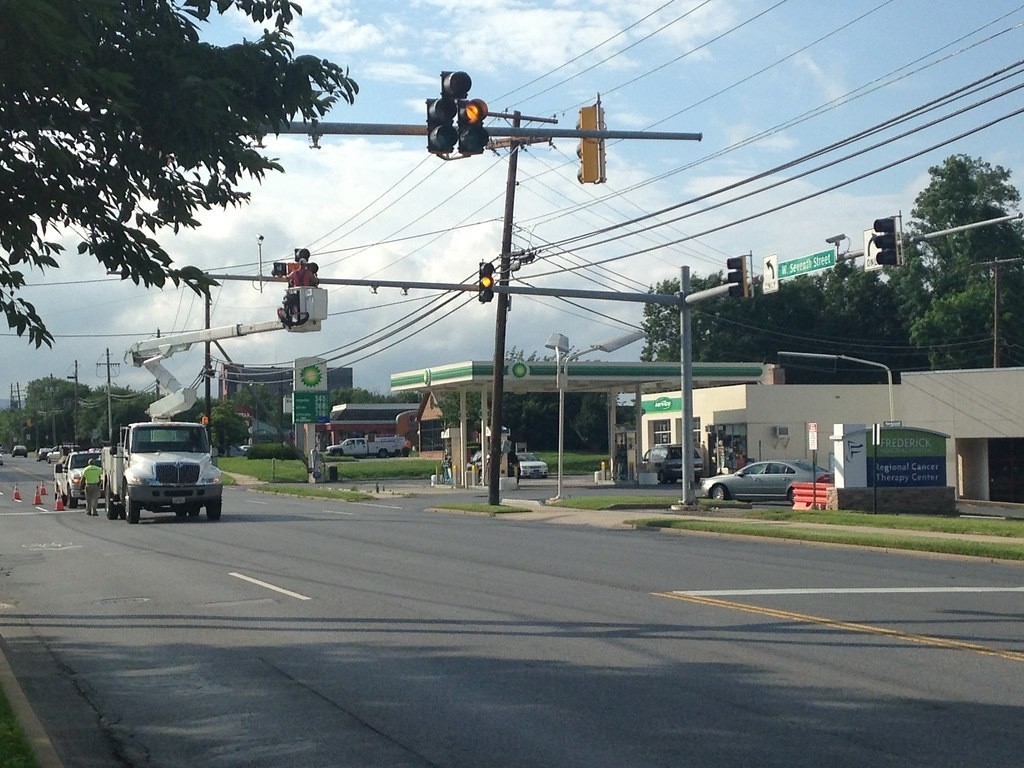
100,287,329,525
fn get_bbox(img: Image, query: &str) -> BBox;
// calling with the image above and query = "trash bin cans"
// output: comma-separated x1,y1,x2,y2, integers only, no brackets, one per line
329,466,338,482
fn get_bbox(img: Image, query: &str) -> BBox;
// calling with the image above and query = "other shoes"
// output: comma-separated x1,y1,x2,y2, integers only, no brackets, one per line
86,512,91,515
93,513,99,516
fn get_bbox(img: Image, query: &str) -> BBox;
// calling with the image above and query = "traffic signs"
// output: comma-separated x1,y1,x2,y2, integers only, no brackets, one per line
763,253,779,294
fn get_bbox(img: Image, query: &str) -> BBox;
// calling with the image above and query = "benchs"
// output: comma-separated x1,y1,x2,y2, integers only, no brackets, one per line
133,441,200,450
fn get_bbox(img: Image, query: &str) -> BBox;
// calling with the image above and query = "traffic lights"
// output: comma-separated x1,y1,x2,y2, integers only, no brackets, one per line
457,99,490,155
479,262,494,303
576,106,599,184
727,256,749,298
425,98,459,153
287,248,320,287
873,216,900,267
440,71,472,100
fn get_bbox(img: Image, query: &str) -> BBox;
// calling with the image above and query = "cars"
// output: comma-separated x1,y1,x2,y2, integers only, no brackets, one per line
0,445,104,509
12,445,28,458
701,460,834,506
516,453,547,479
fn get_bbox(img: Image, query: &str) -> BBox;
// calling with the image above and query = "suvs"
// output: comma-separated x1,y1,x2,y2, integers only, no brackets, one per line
642,444,704,484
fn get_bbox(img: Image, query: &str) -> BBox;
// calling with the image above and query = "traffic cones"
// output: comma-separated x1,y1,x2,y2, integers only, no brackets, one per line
40,480,48,495
12,483,21,500
32,485,44,505
54,489,65,511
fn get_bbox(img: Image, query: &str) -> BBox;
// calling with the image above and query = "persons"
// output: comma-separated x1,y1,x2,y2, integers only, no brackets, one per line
80,459,103,516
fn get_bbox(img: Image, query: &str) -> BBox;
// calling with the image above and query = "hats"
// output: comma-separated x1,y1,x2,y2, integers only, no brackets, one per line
88,458,95,465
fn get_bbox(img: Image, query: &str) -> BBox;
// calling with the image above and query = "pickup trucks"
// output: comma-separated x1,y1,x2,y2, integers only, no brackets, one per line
326,438,396,458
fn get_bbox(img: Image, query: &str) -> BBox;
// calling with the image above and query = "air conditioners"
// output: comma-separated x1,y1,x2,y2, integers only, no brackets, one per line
773,424,792,439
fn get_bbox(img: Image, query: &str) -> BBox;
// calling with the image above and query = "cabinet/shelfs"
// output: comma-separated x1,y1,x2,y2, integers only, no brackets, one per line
710,437,746,478
